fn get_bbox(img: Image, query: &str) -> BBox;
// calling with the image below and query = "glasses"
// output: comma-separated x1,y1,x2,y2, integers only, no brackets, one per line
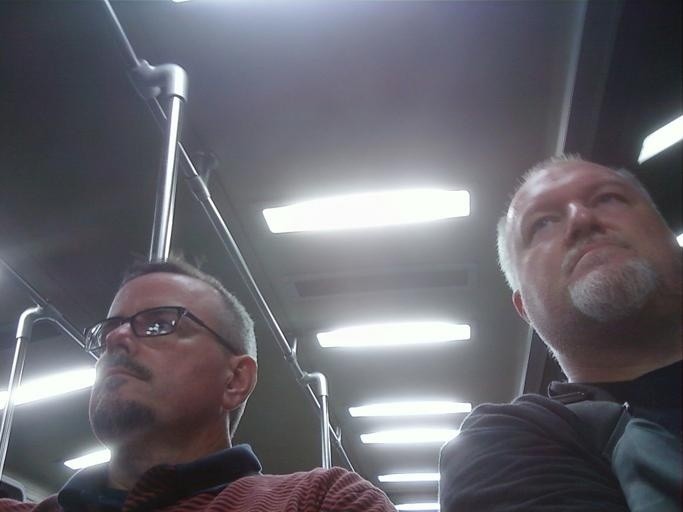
81,305,241,356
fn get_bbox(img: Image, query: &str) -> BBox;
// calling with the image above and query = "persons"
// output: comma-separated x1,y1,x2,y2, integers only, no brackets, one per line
437,151,682,512
0,254,398,512
